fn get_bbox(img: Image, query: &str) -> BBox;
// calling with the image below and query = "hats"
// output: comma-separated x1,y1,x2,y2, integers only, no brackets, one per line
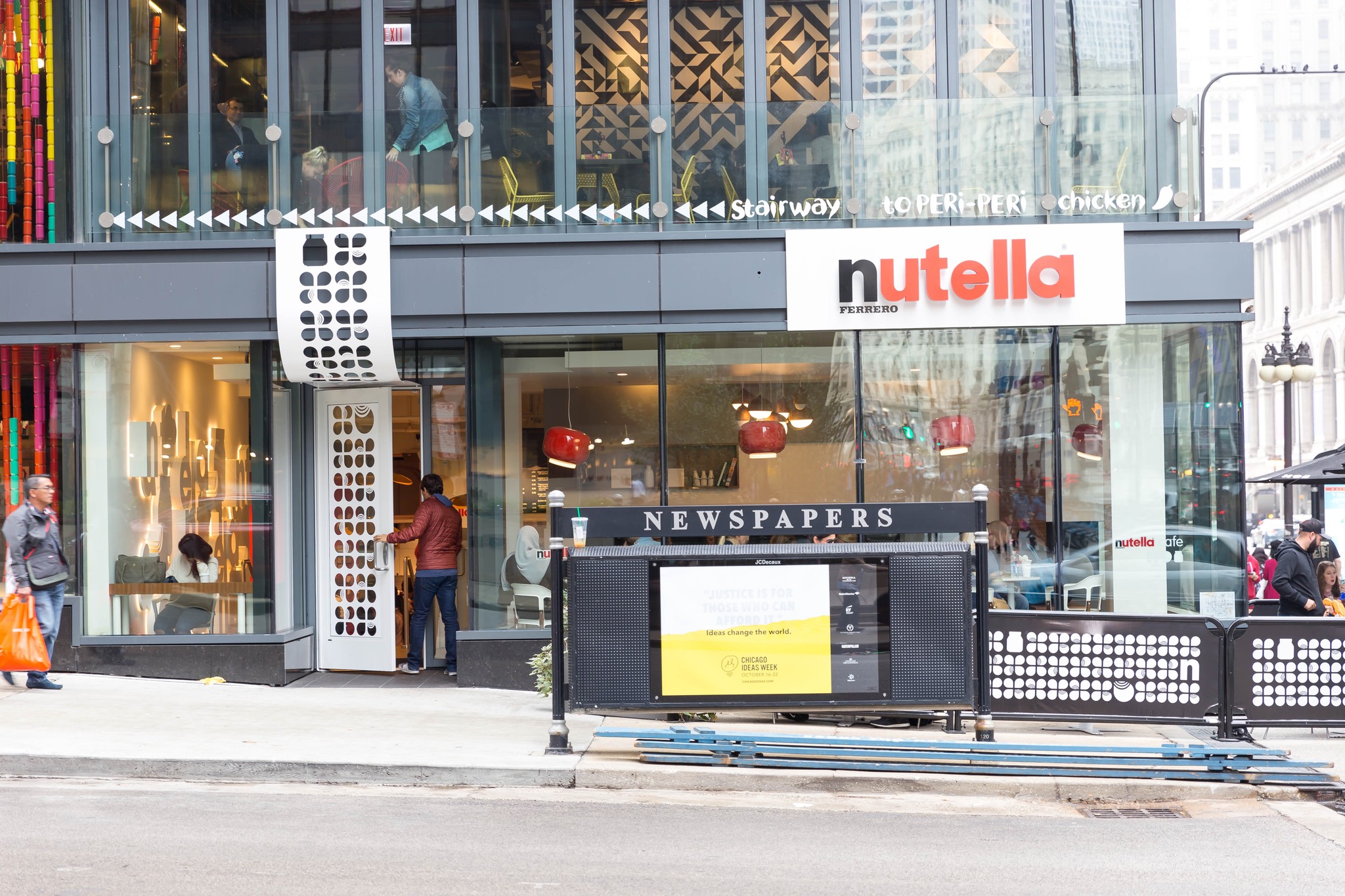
1299,518,1332,539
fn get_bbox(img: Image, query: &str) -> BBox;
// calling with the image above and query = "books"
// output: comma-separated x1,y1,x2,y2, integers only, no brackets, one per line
717,458,737,486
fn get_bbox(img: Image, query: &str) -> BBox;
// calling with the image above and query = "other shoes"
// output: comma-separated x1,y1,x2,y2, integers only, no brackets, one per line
846,625,865,631
26,679,63,689
2,671,14,685
836,626,846,631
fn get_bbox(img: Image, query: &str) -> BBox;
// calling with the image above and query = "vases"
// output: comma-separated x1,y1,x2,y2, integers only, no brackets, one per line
644,465,654,488
693,470,713,486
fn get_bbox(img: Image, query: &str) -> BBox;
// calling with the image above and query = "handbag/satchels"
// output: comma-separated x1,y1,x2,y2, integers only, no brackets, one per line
1255,578,1268,599
1019,542,1055,604
116,555,166,583
26,544,68,591
0,594,51,672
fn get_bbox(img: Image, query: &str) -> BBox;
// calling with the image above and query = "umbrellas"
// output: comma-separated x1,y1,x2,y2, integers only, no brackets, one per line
1245,443,1345,488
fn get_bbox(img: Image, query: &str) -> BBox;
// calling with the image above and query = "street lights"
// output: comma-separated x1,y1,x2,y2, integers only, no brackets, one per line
1256,307,1317,532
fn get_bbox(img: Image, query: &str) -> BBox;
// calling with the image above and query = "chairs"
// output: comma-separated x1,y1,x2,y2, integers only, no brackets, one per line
528,192,554,227
176,170,257,232
496,156,554,227
576,172,620,210
152,595,217,634
1069,146,1130,214
803,189,841,222
1045,573,1105,610
322,156,410,225
971,587,994,608
636,155,698,225
721,165,780,222
510,583,551,628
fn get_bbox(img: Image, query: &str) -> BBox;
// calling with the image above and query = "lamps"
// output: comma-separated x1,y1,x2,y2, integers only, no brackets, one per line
926,416,977,456
621,425,635,445
1071,419,1103,460
542,335,591,469
730,331,813,459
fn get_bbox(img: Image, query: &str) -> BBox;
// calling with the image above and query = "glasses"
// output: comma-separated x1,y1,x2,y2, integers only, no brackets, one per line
229,107,244,112
29,487,55,492
212,76,218,86
818,538,838,543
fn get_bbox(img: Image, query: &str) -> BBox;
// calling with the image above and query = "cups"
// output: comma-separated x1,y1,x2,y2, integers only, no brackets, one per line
571,517,588,547
1021,560,1032,577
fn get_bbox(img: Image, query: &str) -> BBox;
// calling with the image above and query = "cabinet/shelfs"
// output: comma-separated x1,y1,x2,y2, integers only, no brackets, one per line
546,442,741,491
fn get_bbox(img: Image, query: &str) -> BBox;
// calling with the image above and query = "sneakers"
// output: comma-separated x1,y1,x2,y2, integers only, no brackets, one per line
444,669,457,675
781,713,809,722
870,717,911,728
399,663,419,674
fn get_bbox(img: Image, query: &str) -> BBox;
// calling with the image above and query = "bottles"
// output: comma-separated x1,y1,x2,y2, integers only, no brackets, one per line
708,470,714,486
693,470,700,485
644,465,654,487
701,471,707,486
1010,551,1019,578
1015,550,1021,576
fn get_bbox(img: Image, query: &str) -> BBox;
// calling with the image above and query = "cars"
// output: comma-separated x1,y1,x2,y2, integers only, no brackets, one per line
1250,513,1311,548
984,523,1247,623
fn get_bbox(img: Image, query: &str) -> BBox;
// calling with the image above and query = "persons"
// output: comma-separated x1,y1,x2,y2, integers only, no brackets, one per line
498,525,552,620
627,537,661,546
886,467,1099,562
169,61,508,226
374,475,462,675
1,474,69,690
1247,518,1345,617
900,520,1029,609
153,533,219,635
705,534,857,545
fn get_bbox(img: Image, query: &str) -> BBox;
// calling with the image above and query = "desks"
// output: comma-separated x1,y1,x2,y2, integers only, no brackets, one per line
576,157,642,208
1001,576,1040,609
109,582,253,635
768,164,829,221
